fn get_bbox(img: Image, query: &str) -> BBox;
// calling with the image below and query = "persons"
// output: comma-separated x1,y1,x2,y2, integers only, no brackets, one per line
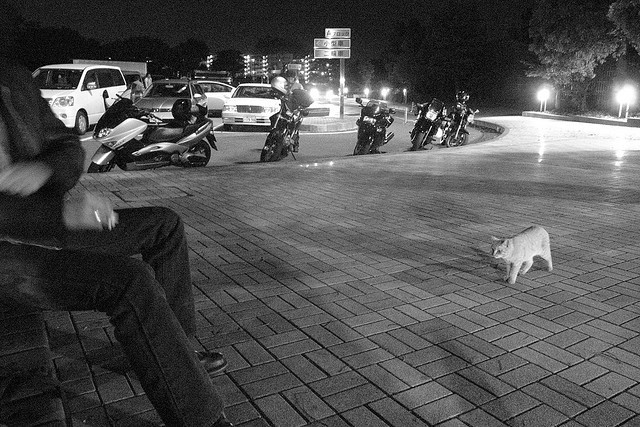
0,55,233,426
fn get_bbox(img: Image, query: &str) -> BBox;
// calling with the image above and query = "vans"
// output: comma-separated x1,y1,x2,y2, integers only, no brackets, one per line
121,69,143,87
30,63,127,135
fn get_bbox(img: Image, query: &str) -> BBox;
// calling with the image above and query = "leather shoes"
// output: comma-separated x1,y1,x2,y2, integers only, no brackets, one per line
198,350,227,371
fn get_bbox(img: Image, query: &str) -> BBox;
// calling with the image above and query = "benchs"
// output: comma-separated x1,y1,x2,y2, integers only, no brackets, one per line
1,294,65,425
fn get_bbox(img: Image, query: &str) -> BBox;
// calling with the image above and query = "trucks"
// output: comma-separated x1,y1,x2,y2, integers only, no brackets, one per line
74,58,153,79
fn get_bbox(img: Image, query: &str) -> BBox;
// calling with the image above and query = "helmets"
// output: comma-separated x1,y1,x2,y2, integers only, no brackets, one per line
455,91,469,103
272,75,289,94
171,99,191,118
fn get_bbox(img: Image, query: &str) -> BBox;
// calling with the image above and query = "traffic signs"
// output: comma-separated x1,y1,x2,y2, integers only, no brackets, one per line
325,28,350,38
314,39,350,47
314,48,351,58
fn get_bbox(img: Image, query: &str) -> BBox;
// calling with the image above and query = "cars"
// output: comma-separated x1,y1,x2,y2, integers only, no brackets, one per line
133,79,208,128
305,81,335,103
194,79,236,117
221,83,283,130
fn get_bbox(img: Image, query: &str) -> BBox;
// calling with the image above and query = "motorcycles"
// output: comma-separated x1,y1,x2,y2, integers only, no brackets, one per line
410,96,447,151
261,88,314,162
353,97,397,155
439,90,480,147
86,88,219,172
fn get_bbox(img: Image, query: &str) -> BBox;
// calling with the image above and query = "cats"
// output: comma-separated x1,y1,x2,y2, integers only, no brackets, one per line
488,223,554,285
